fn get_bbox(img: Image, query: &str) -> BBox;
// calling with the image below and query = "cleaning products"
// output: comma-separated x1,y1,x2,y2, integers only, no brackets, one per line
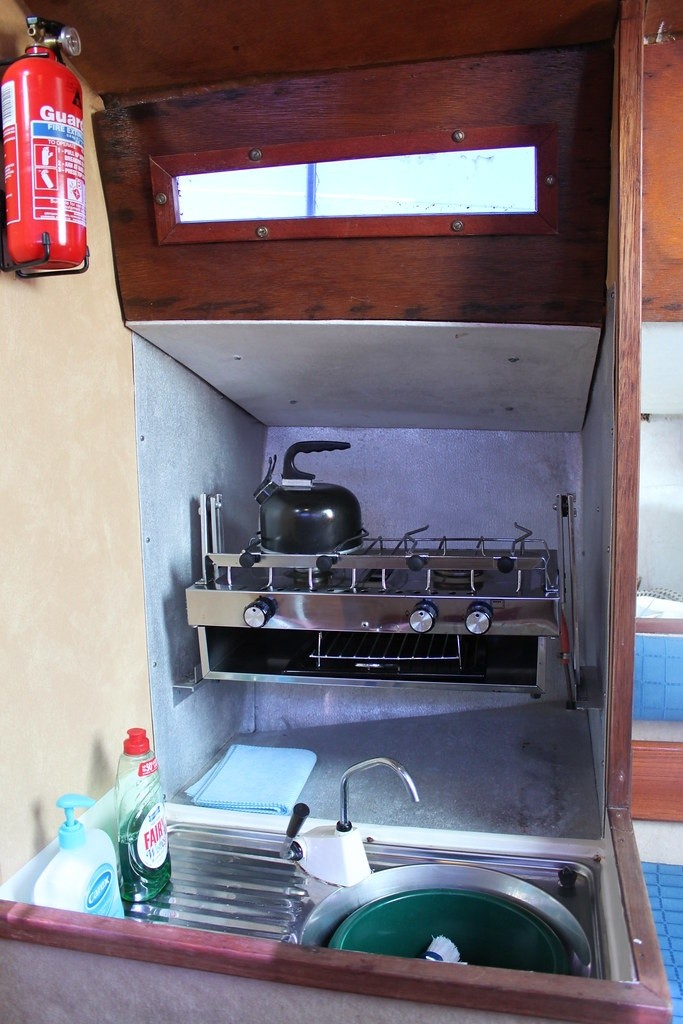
111,727,174,907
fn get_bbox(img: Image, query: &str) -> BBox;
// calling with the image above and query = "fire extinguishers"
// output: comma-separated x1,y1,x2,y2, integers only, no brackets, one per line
0,12,92,280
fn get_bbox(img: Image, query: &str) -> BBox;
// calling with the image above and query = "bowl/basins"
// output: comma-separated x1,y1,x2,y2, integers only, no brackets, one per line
331,886,566,977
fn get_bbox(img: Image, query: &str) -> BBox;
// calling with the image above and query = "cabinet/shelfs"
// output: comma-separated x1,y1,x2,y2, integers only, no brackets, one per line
0,0,683,1024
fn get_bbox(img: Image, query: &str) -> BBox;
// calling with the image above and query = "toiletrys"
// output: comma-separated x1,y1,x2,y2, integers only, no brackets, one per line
28,794,129,924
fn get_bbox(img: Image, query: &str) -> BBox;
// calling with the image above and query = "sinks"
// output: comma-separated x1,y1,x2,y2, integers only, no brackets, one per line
298,852,639,987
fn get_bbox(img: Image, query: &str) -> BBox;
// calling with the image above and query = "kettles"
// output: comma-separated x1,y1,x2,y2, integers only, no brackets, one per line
253,440,364,556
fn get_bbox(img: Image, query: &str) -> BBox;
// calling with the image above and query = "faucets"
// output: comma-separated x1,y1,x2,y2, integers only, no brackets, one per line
336,755,421,835
